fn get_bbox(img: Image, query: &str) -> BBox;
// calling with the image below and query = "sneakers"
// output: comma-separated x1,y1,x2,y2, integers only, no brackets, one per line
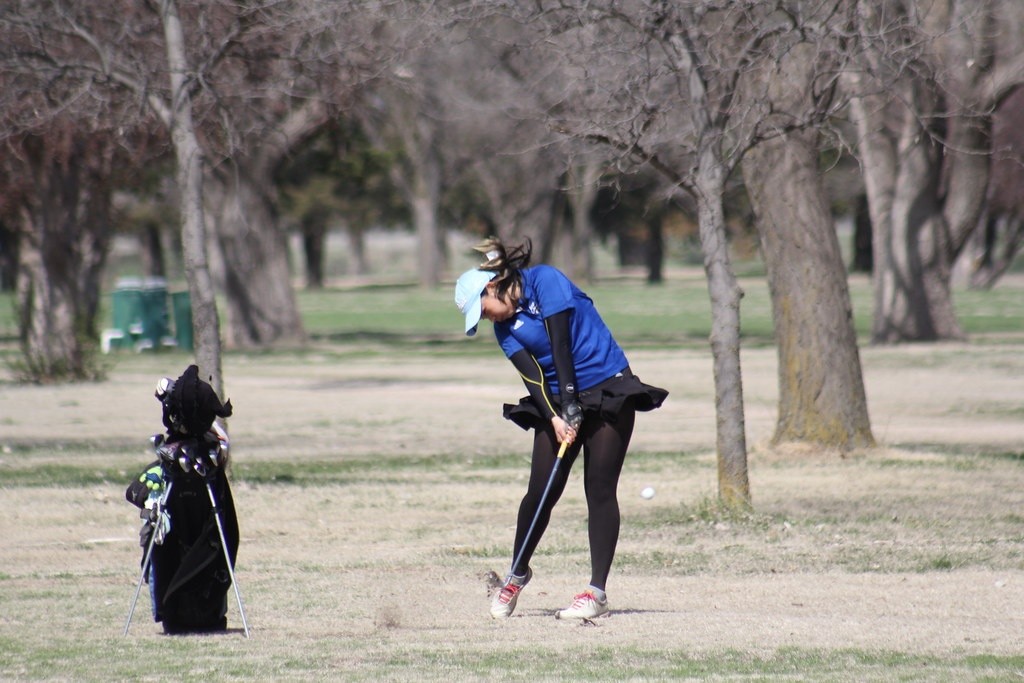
491,565,532,620
555,588,611,619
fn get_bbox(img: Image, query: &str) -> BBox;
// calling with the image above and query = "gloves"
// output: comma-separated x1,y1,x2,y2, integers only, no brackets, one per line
562,401,584,430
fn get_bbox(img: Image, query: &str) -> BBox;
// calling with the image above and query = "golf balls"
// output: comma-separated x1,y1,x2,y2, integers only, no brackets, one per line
641,487,654,499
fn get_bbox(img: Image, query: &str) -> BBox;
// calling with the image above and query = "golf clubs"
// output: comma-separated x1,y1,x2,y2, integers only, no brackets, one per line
488,405,581,588
149,433,230,476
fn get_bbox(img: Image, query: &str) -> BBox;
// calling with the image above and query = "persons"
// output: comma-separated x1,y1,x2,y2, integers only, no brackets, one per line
453,235,671,621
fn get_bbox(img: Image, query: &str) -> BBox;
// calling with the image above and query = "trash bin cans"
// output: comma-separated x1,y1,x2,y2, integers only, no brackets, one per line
101,278,173,353
172,290,192,353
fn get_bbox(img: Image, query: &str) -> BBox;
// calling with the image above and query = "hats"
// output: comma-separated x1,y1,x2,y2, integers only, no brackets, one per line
455,268,496,337
